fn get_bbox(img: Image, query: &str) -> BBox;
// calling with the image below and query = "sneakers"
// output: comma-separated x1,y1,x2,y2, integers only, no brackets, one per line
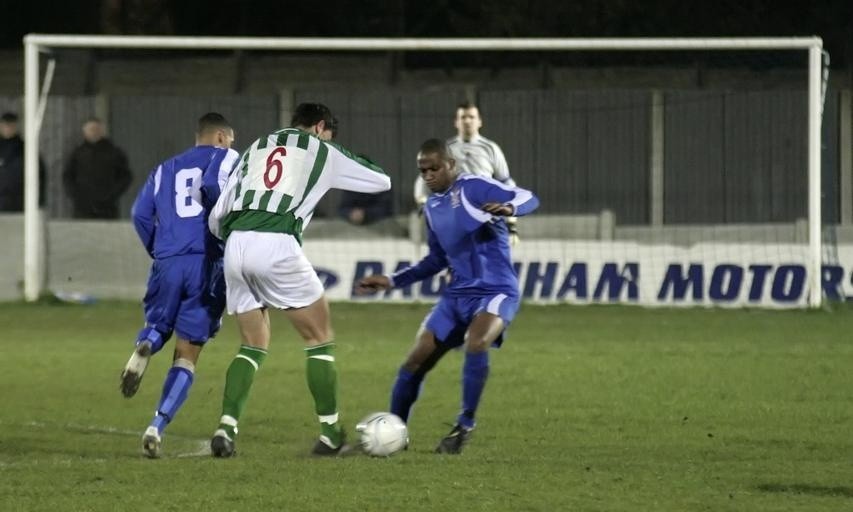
142,426,161,458
211,435,235,458
119,340,151,397
434,422,476,455
314,425,347,455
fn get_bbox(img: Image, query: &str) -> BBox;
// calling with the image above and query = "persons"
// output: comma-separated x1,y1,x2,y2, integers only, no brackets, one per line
0,111,47,212
61,116,132,219
351,137,540,455
207,99,391,457
118,111,241,459
340,155,392,226
412,104,520,282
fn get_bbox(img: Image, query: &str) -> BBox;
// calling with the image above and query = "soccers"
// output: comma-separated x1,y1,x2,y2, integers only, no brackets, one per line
356,410,409,460
